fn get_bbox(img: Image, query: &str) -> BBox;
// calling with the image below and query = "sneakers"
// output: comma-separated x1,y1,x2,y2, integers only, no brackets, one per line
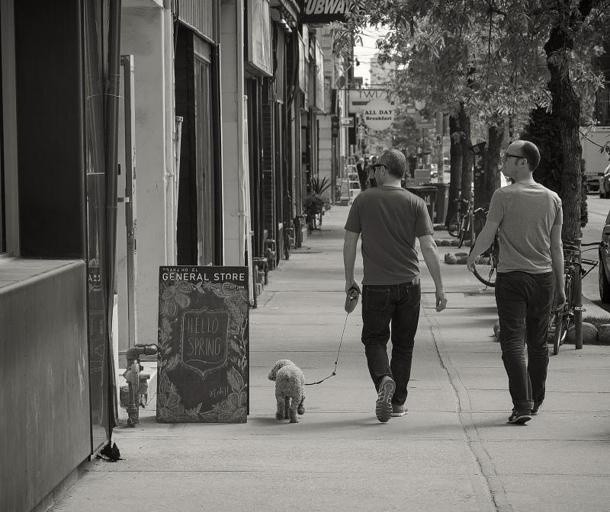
391,402,409,417
507,407,532,424
376,376,396,422
531,399,543,415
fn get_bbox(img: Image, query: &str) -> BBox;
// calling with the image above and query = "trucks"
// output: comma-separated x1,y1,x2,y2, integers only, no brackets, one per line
579,125,609,191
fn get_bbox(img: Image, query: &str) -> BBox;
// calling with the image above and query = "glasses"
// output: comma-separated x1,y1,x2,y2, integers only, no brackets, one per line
372,164,386,173
505,152,523,162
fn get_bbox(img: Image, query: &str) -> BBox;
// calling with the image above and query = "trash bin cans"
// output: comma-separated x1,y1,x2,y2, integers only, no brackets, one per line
405,182,445,225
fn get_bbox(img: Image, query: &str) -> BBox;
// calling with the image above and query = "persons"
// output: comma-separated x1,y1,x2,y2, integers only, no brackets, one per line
466,138,570,427
341,147,449,425
355,154,380,191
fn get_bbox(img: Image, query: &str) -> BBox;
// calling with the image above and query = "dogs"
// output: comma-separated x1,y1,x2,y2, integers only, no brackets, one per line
268,358,306,424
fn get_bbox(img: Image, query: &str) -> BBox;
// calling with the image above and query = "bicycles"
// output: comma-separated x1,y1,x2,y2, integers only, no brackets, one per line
468,208,499,287
447,194,472,248
548,242,599,354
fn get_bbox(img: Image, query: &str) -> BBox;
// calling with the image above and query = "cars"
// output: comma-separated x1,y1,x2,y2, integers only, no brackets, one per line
599,211,610,303
598,163,610,197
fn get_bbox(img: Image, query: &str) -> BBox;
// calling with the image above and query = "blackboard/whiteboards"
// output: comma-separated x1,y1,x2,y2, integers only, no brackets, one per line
155,265,250,424
